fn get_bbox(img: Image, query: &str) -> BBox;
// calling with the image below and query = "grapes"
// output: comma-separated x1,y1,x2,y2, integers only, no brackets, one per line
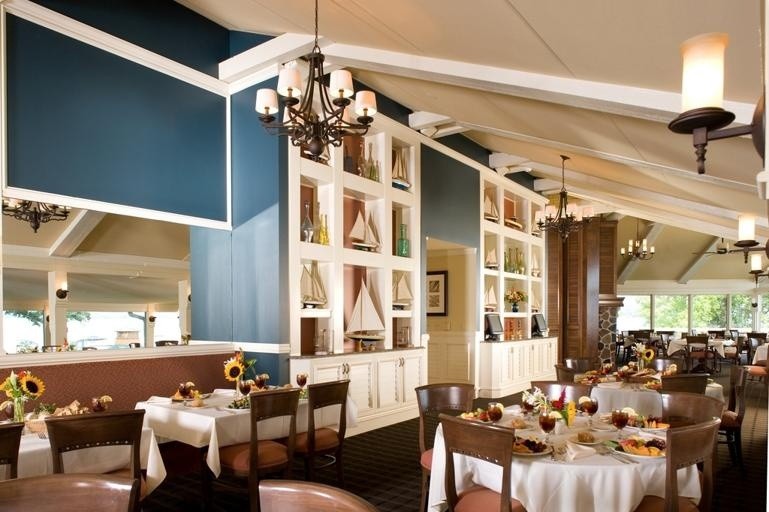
522,439,547,453
645,439,666,449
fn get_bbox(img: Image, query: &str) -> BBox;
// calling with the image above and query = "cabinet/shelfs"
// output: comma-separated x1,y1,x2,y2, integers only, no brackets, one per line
308,349,425,431
287,81,427,361
479,169,560,399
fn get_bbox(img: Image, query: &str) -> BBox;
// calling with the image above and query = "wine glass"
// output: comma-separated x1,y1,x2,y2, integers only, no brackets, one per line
452,388,671,465
171,372,309,411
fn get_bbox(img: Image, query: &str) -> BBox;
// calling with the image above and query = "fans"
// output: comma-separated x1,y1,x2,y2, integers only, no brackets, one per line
692,237,744,257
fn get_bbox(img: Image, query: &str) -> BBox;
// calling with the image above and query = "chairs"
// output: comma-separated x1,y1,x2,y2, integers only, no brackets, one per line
0,330,769,512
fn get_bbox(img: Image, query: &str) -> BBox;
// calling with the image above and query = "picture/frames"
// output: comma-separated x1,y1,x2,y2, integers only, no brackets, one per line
426,270,448,316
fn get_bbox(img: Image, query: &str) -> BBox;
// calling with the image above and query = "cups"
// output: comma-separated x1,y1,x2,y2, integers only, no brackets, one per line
3,403,15,419
93,397,113,413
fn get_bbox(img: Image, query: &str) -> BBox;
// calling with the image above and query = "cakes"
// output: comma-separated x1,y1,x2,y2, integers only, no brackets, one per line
578,431,594,441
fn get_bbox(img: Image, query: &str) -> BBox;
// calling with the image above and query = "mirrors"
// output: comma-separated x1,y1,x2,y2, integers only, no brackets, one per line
2,195,192,355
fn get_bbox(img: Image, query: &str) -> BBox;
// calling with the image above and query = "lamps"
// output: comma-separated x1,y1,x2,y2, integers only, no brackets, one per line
748,254,769,287
2,196,72,234
620,218,655,263
535,155,594,242
729,213,769,263
666,0,765,175
256,1,377,162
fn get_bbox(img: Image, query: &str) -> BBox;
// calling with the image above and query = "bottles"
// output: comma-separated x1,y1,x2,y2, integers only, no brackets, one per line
398,223,411,257
299,196,331,244
354,140,382,182
504,247,525,275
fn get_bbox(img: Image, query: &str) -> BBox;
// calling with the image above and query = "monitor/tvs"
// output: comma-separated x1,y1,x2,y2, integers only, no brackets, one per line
486,314,503,335
534,314,547,332
